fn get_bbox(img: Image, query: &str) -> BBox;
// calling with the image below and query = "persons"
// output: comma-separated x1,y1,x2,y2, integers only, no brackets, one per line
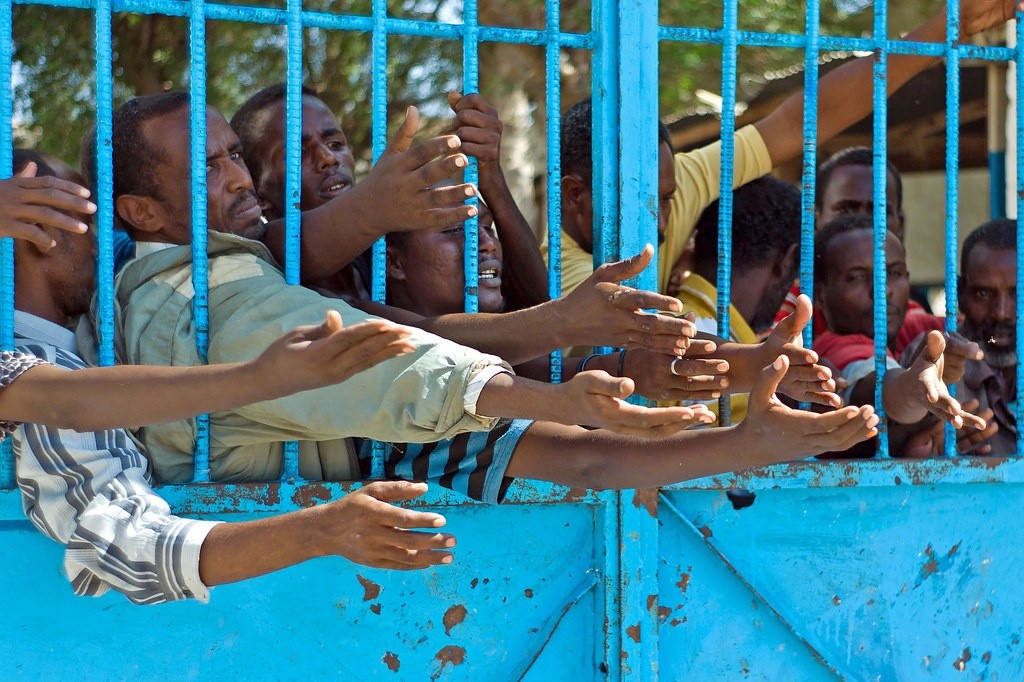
539,0,1024,358
0,82,1016,607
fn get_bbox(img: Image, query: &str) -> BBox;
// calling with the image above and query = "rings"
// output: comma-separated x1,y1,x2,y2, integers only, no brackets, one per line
670,358,680,375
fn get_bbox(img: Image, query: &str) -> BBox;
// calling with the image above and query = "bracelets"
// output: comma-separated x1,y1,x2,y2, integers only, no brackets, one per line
576,354,600,372
617,350,627,377
